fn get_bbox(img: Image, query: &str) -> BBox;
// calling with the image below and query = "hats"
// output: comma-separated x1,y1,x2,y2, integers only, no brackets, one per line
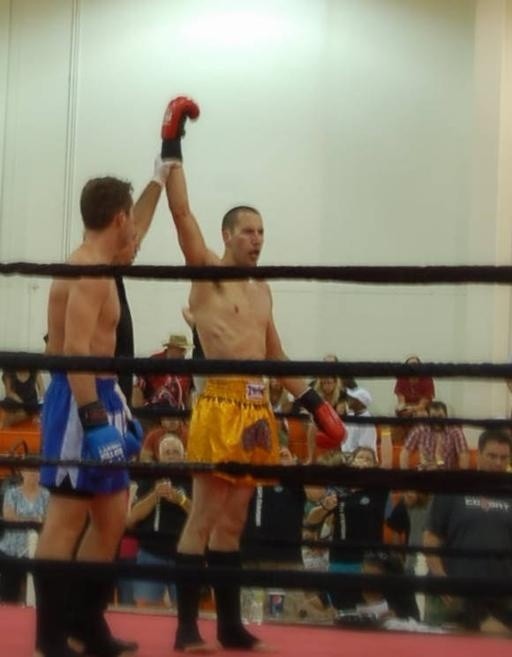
159,333,198,350
345,386,375,409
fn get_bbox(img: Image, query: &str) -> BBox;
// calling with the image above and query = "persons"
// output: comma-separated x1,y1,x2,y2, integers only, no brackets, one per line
28,175,146,655
66,159,184,657
158,96,347,649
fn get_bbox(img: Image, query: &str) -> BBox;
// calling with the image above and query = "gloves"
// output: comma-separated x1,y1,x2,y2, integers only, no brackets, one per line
77,398,130,480
293,384,349,451
150,154,167,188
158,94,201,166
